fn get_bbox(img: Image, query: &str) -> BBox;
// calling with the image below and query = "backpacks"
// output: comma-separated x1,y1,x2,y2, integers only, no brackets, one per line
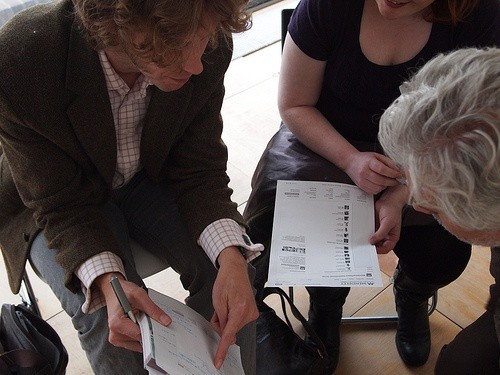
0,304,68,375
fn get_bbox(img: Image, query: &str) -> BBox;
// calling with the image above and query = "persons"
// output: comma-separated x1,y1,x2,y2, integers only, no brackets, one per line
378,47,500,375
243,0,500,375
0,0,265,375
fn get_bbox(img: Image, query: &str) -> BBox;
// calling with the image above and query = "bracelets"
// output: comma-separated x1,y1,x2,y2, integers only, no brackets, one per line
395,176,409,186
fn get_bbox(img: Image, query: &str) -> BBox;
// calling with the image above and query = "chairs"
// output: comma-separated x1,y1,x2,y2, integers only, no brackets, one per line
280,8,439,325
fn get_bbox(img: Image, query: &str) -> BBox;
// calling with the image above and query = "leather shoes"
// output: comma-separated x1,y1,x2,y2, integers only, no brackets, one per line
395,292,430,368
305,308,342,375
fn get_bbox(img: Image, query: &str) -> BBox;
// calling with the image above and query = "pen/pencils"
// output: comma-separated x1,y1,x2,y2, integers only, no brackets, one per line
109,276,142,347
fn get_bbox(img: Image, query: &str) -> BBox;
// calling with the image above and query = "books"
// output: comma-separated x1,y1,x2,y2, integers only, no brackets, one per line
138,288,169,375
